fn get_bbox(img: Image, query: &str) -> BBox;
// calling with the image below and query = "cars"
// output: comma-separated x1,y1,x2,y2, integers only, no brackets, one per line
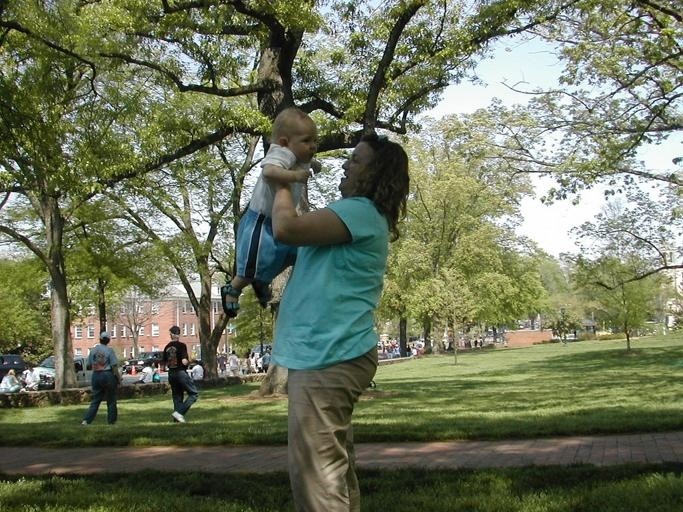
125,352,170,374
0,355,27,383
22,354,123,389
242,344,272,375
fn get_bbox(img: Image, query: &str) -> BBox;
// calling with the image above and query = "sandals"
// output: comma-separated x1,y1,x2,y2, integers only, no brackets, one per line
221,284,240,318
251,277,274,309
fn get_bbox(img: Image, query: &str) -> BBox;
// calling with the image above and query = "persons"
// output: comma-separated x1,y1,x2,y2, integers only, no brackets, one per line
217,348,271,376
160,326,199,424
81,332,122,425
221,107,323,318
132,358,205,384
473,335,483,350
0,364,43,393
262,133,410,512
381,337,426,359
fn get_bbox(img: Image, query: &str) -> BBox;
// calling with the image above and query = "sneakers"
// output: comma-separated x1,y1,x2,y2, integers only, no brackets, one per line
171,411,186,424
81,420,88,426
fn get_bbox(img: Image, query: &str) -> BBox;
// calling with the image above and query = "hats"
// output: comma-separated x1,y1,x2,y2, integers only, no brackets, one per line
169,325,181,335
100,331,111,341
